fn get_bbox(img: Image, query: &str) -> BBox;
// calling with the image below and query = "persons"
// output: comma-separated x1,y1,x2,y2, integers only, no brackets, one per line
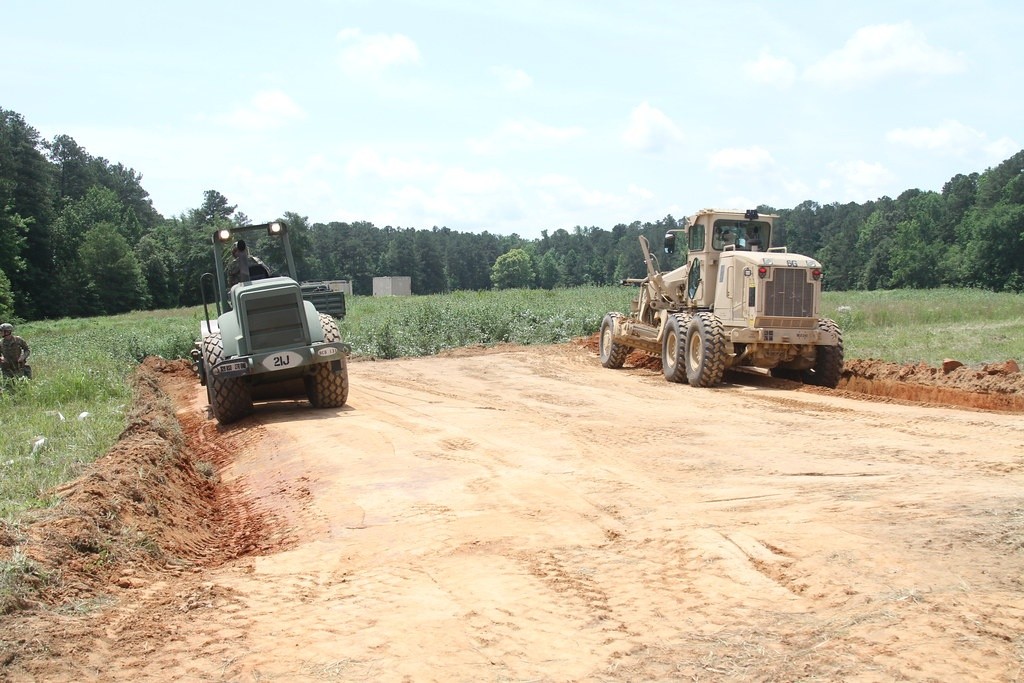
226,240,272,286
0,323,32,381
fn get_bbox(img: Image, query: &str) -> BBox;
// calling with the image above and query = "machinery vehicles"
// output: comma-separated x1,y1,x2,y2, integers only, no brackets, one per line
598,209,845,389
189,222,352,423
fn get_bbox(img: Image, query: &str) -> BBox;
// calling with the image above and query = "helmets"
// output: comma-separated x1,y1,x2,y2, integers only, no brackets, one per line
231,241,249,258
720,231,737,241
0,323,13,331
